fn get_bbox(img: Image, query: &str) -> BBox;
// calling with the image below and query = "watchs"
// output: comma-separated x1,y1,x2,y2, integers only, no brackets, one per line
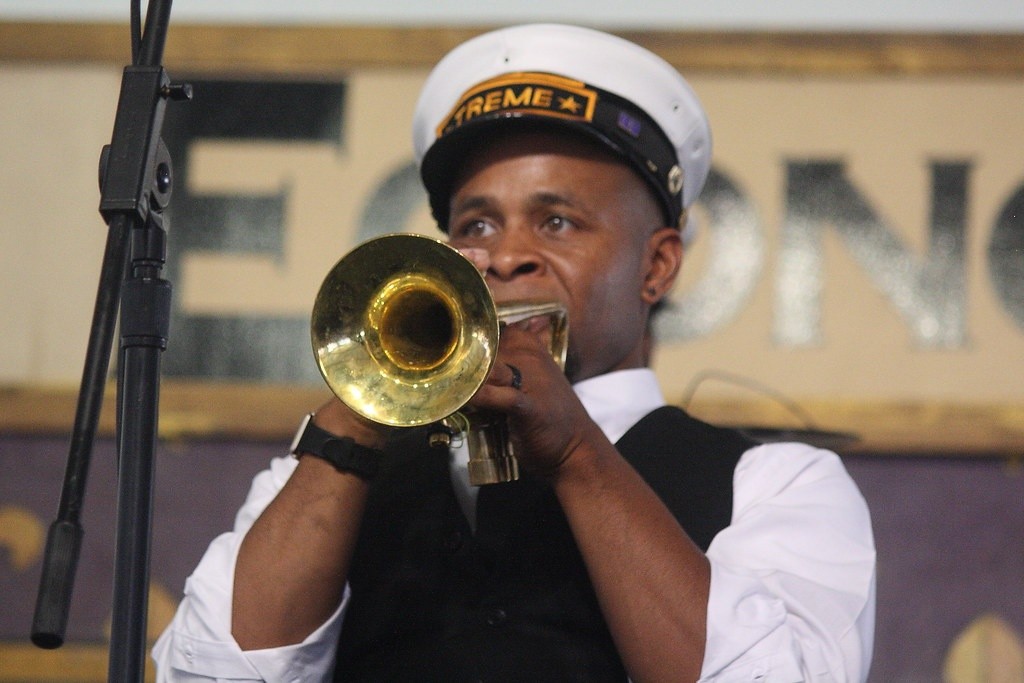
288,413,389,486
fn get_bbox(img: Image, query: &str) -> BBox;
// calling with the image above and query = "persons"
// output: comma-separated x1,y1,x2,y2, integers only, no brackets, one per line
150,24,878,683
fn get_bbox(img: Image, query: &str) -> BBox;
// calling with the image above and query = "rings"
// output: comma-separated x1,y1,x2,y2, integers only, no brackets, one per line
505,362,522,389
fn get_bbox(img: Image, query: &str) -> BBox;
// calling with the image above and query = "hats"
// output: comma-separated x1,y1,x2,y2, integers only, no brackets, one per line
412,23,712,232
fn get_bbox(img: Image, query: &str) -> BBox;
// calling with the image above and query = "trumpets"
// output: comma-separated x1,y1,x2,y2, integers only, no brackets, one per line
310,230,570,486
